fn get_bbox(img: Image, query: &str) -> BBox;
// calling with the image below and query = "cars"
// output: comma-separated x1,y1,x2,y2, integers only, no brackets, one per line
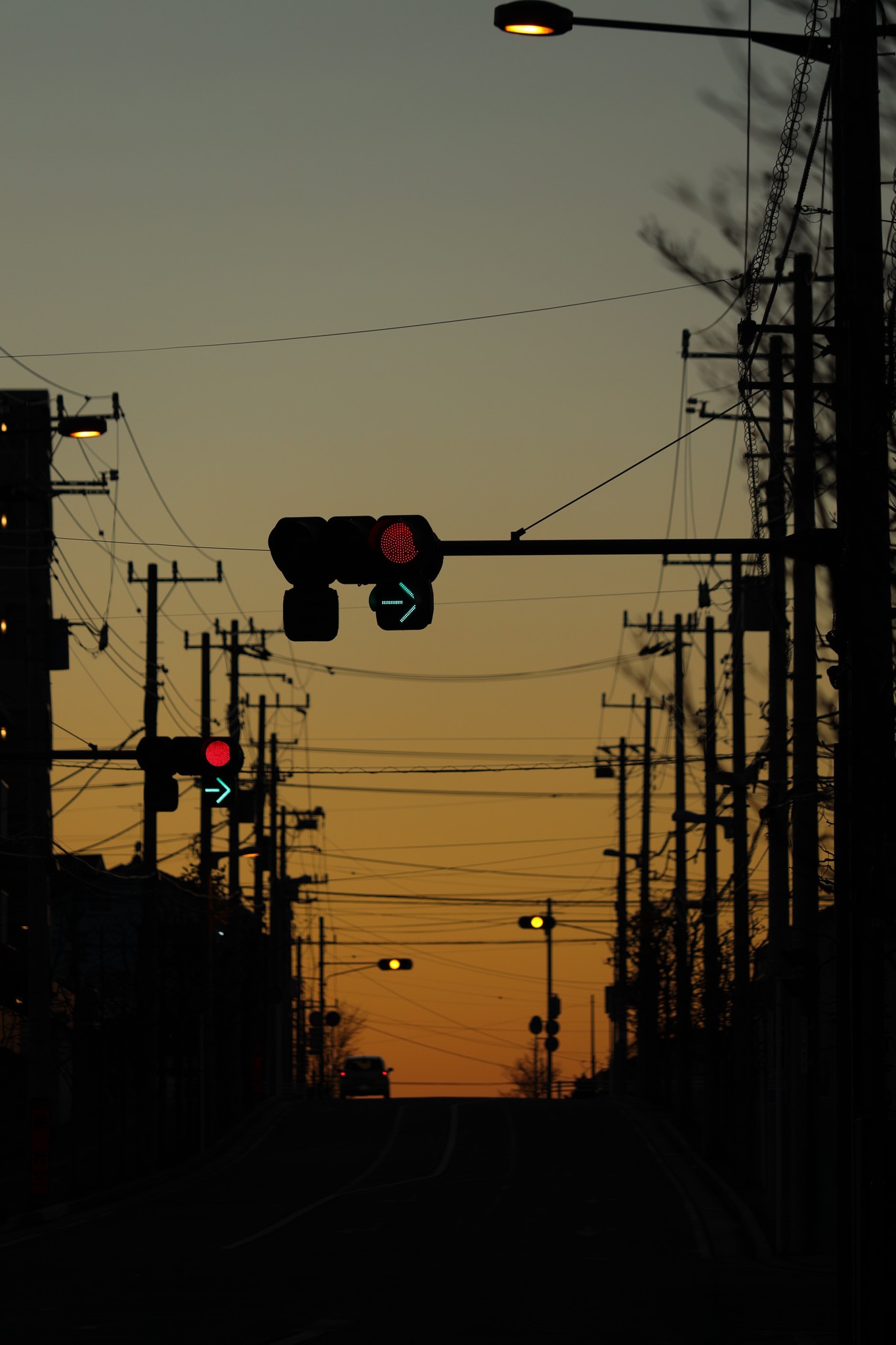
336,1056,394,1099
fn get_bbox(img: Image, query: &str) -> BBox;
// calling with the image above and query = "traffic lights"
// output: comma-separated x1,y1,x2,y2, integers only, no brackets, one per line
200,777,242,812
378,958,413,970
267,514,447,585
135,735,246,777
367,582,433,632
517,914,556,931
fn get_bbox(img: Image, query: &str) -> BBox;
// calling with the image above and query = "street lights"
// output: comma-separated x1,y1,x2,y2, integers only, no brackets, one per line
2,389,107,1258
496,1,890,1343
